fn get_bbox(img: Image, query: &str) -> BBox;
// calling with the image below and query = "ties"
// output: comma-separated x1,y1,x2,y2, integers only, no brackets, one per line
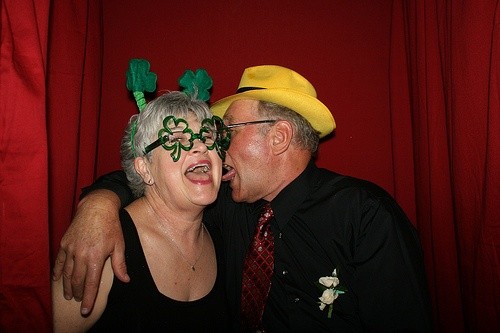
239,202,274,333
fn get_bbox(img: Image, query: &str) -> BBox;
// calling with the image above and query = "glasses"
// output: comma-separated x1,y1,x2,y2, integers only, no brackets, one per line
142,114,231,161
218,119,276,139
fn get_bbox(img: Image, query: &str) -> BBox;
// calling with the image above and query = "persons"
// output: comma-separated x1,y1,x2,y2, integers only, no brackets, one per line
52,65,429,333
50,89,246,333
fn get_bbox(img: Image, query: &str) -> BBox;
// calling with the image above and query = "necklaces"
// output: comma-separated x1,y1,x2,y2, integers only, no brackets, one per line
144,196,206,272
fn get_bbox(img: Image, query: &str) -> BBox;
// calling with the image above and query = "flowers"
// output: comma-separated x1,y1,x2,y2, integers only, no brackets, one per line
317,269,345,319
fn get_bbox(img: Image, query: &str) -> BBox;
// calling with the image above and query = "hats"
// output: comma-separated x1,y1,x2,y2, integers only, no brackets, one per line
210,64,337,138
